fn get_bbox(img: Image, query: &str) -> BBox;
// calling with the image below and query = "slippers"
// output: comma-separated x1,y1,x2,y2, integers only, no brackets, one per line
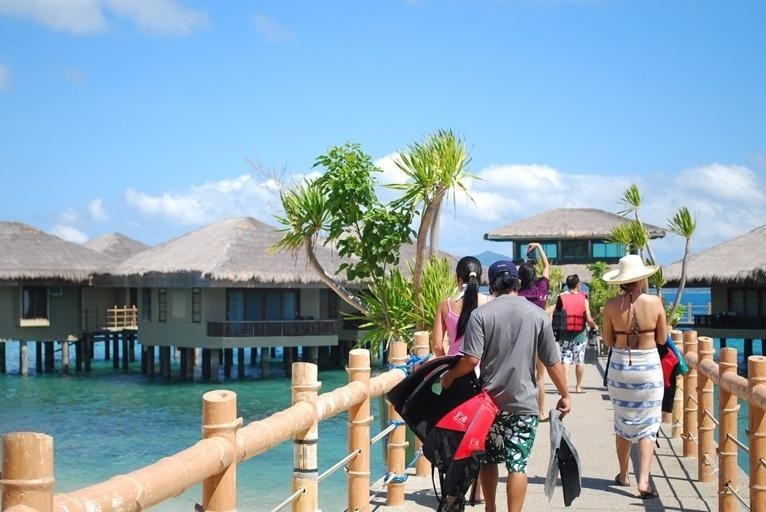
615,472,632,487
638,488,659,499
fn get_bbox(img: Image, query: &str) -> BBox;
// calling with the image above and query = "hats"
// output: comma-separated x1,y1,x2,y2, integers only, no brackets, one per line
487,258,520,285
602,253,661,285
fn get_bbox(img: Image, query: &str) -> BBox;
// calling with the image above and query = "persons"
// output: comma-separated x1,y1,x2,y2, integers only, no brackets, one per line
440,260,572,512
599,253,669,501
552,273,599,394
516,241,552,422
431,255,495,504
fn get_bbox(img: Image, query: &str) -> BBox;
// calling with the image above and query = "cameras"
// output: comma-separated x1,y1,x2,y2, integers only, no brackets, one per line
526,245,538,266
